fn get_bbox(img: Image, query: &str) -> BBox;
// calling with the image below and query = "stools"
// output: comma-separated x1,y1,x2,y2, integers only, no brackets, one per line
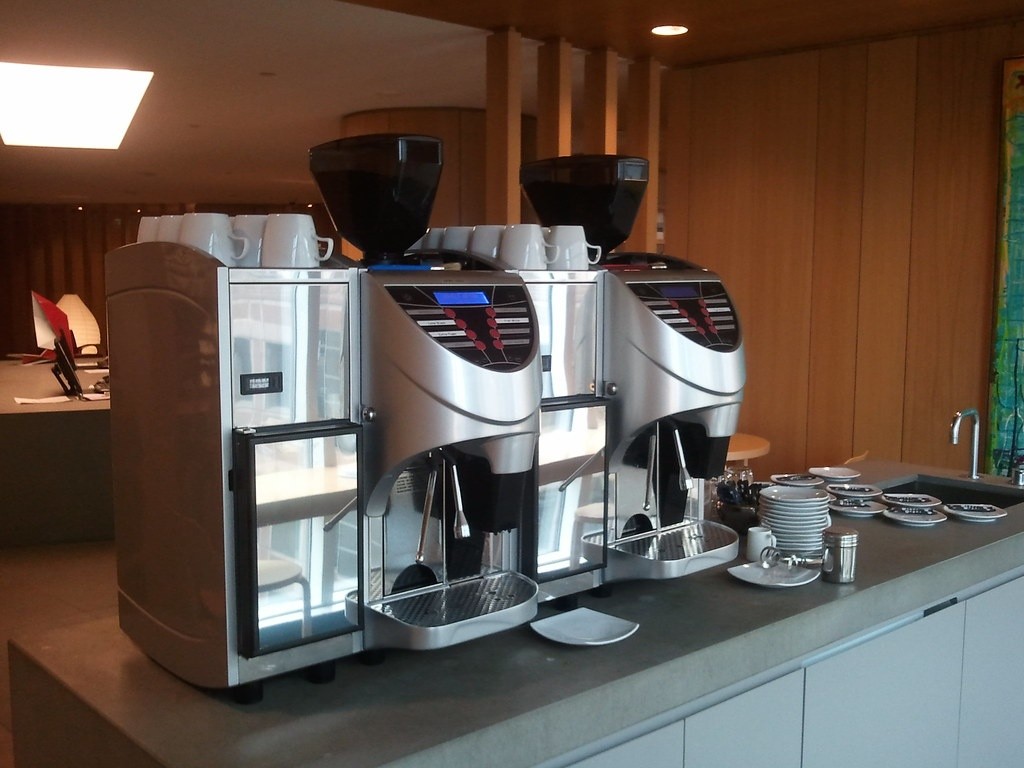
570,502,617,570
257,545,312,639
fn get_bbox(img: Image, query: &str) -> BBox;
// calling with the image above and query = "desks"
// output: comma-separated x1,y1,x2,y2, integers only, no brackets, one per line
256,428,605,608
0,359,113,544
726,433,772,467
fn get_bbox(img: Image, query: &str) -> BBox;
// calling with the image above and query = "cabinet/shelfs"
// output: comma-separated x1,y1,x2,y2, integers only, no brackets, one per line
535,564,1024,768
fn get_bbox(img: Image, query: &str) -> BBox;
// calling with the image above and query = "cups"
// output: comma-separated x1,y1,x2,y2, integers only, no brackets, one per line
746,527,777,561
138,213,333,269
704,464,753,519
405,222,601,271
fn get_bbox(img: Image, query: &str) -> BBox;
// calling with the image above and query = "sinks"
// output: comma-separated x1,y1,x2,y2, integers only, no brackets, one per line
869,473,1024,512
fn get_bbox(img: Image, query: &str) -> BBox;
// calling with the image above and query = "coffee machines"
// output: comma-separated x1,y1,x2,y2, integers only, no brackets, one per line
516,153,746,601
105,135,542,703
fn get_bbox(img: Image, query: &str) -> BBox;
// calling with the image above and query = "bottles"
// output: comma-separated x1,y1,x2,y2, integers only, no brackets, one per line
820,524,859,585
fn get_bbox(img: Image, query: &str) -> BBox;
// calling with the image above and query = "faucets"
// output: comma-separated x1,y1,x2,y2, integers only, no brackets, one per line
948,408,981,479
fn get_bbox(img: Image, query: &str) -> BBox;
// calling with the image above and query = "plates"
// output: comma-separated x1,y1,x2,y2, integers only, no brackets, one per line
727,561,821,588
530,606,639,646
759,466,1007,549
